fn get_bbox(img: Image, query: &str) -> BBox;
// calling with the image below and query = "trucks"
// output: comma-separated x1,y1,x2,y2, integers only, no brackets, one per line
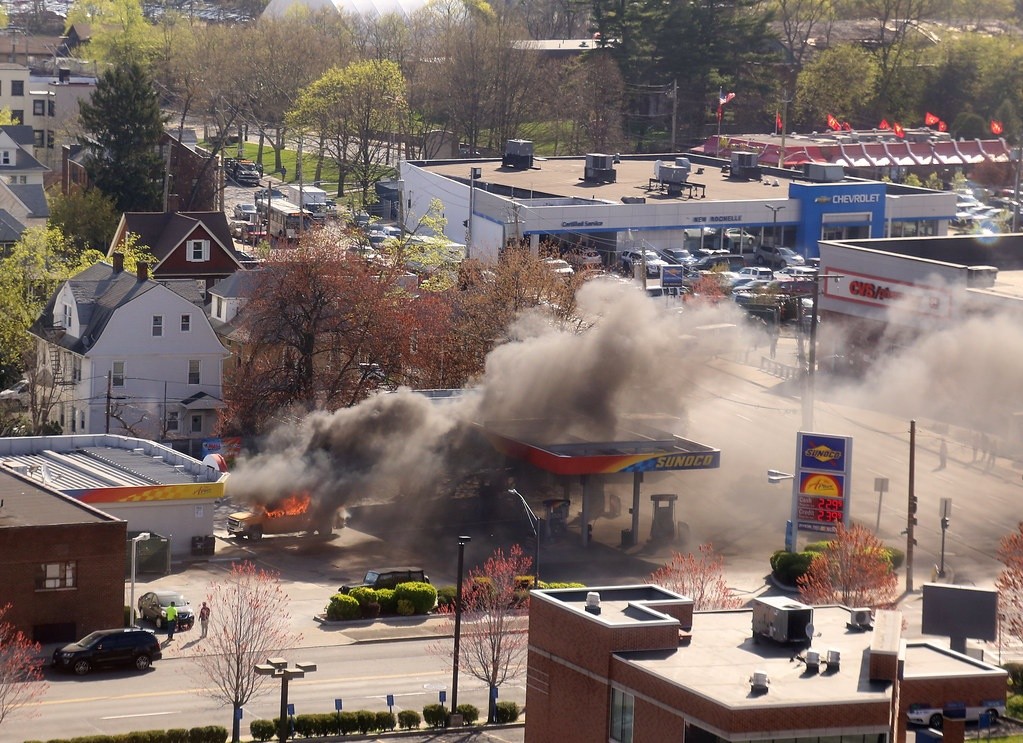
224,157,261,186
287,184,327,228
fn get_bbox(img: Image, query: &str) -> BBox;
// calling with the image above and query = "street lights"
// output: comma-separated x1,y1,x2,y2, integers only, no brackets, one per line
451,535,472,715
131,532,151,628
508,488,541,589
765,203,786,280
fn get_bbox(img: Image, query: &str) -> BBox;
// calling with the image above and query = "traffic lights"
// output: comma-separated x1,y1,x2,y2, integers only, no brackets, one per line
942,518,949,529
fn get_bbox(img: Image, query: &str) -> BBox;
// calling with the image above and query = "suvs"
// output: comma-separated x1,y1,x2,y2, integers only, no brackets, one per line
336,566,430,598
51,627,163,677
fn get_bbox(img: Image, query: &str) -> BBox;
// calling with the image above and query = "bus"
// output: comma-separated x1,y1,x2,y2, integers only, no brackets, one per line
256,198,313,245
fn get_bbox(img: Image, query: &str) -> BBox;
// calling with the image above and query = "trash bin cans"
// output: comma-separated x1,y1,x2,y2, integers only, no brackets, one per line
205,536,215,556
622,529,633,545
192,536,202,556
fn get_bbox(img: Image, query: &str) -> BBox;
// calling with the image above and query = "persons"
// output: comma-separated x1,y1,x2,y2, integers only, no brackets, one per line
198,601,211,639
770,336,776,358
281,166,287,182
166,601,178,641
940,431,997,468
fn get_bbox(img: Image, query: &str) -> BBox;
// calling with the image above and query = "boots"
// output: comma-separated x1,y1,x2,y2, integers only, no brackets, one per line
167,636,175,641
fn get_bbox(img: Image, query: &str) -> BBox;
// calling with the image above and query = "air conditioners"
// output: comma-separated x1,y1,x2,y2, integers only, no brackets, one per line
851,606,871,625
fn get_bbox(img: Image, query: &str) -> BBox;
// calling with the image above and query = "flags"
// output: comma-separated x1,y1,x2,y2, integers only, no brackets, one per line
827,112,1004,139
775,112,783,128
715,92,736,121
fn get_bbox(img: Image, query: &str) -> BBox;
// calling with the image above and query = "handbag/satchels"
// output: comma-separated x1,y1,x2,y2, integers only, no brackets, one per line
201,609,206,620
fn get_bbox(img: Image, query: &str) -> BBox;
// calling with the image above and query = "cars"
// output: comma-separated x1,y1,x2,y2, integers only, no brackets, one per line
226,500,339,544
949,184,1023,233
233,203,256,221
906,703,1007,730
240,223,267,245
137,590,195,630
620,226,821,329
544,257,575,279
567,246,603,268
342,210,397,264
229,220,248,239
